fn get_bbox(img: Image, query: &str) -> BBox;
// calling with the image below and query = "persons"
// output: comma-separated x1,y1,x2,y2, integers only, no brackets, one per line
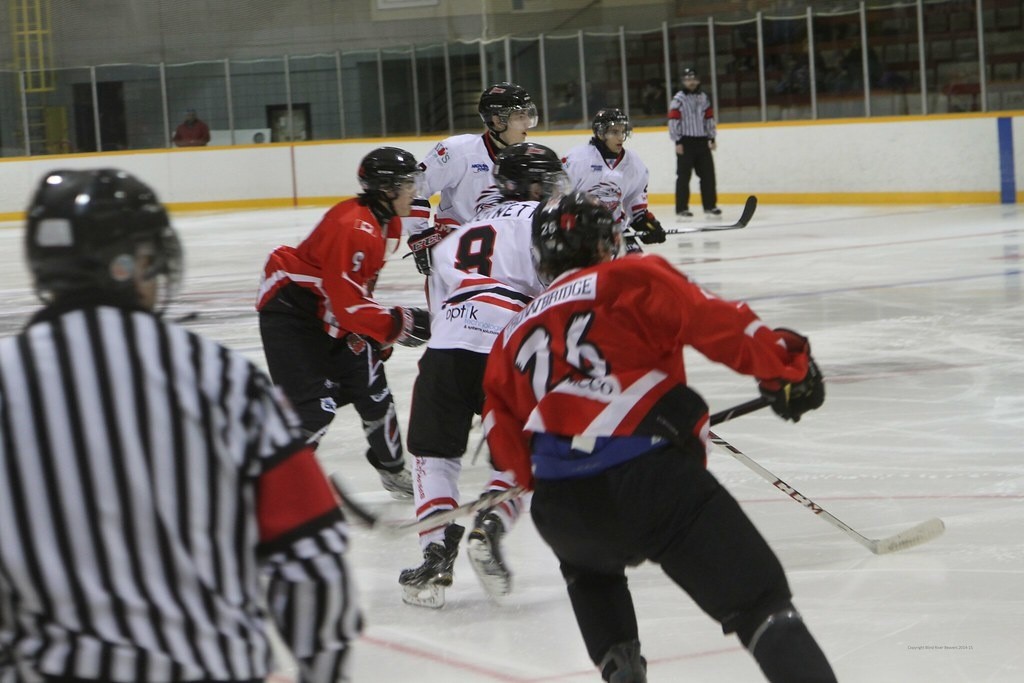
257,147,429,503
396,143,570,609
642,77,666,115
172,109,210,147
668,67,721,216
485,191,839,683
560,108,667,254
400,81,538,275
0,168,363,683
723,32,881,121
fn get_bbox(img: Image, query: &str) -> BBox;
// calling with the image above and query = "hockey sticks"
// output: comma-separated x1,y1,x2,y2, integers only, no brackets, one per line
621,194,759,238
327,393,778,541
708,428,946,555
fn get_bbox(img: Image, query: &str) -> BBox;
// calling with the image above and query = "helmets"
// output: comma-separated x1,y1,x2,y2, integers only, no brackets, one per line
477,82,532,125
493,143,562,198
530,191,622,271
357,147,417,199
25,168,181,316
592,108,629,135
680,68,698,80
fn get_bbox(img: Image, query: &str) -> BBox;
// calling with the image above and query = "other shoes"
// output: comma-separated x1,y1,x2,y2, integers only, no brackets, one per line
677,210,693,217
704,207,721,216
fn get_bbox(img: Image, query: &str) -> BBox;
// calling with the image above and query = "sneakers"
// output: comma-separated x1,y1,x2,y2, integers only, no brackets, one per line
399,523,465,608
467,513,511,597
367,447,414,500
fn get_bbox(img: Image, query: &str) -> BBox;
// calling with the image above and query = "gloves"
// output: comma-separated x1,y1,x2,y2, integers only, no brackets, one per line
630,209,666,245
408,227,443,276
379,306,432,348
756,328,824,423
